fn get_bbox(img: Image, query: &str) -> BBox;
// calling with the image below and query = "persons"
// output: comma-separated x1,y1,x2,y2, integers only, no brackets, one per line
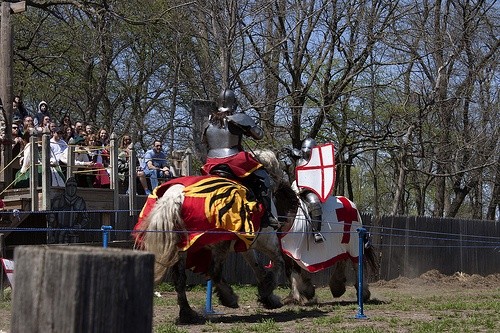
0,96,76,188
199,88,280,228
140,140,174,191
117,135,151,195
71,122,112,189
289,137,324,243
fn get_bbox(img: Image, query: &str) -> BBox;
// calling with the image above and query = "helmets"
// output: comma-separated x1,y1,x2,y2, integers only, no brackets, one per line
301,138,317,152
217,87,237,113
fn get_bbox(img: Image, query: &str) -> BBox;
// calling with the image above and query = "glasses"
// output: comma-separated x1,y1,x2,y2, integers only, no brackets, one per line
76,143,85,146
12,127,19,129
57,132,62,134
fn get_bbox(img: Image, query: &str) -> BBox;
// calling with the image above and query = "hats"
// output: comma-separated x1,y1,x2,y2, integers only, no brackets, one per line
54,127,63,132
74,136,85,144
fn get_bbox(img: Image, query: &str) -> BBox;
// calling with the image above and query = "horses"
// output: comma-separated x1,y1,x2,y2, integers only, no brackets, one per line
273,178,379,308
132,148,284,327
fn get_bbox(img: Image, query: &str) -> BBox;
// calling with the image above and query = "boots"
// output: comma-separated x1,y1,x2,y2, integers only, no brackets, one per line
259,195,278,227
311,215,322,242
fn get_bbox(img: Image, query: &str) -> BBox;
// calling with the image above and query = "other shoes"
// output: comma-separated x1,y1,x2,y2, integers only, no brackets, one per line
145,189,151,195
126,190,129,195
